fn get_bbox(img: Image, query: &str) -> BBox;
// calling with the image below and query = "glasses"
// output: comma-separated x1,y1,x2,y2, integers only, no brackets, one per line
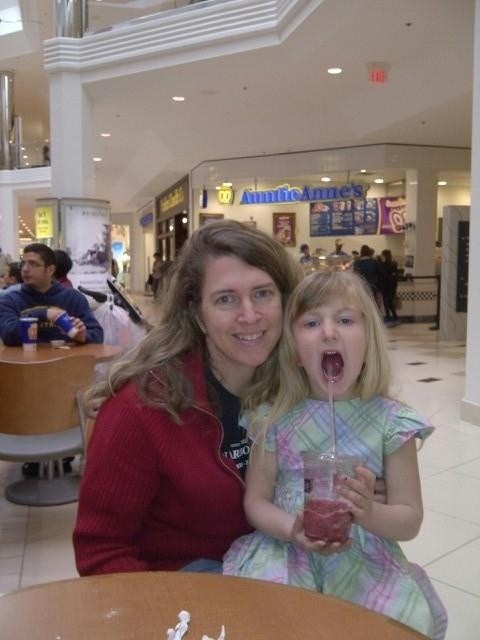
20,260,46,268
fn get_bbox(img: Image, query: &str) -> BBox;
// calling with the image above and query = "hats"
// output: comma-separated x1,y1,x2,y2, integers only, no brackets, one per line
335,239,344,246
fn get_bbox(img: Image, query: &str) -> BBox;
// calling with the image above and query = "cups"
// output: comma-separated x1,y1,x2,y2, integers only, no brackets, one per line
20,318,38,351
53,311,80,338
301,452,369,543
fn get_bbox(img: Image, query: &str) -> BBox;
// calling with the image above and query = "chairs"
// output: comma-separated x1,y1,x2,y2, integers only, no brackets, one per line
0,355,101,476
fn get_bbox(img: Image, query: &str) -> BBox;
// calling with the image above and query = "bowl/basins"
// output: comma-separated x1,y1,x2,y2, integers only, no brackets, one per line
51,341,66,348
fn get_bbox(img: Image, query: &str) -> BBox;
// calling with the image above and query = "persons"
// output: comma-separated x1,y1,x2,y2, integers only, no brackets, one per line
3,262,24,287
326,239,352,271
52,250,74,289
353,245,380,310
299,244,315,266
0,243,104,478
221,269,447,639
352,251,360,257
72,218,306,577
372,250,400,328
111,251,119,277
150,253,165,303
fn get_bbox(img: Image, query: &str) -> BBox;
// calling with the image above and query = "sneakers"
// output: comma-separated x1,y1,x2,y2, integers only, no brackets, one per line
19,462,46,478
53,460,73,474
